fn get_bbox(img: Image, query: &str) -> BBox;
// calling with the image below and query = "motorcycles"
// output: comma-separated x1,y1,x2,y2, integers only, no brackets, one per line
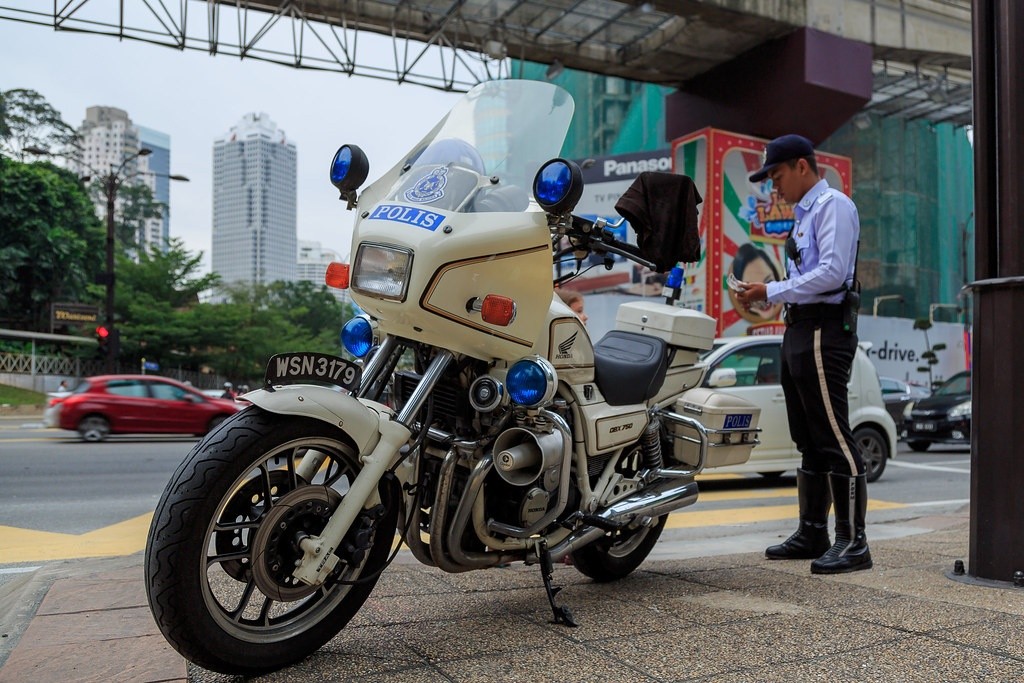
144,80,763,676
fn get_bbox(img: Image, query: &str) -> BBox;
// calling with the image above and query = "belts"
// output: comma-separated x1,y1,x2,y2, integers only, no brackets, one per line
782,303,843,328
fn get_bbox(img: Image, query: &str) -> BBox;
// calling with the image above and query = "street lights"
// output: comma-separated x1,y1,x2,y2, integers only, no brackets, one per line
23,146,190,374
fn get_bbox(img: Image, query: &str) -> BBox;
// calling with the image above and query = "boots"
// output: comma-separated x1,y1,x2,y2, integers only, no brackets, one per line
810,472,874,575
765,468,832,561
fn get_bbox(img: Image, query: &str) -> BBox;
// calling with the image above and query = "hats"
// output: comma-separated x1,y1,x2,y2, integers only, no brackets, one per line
749,135,815,182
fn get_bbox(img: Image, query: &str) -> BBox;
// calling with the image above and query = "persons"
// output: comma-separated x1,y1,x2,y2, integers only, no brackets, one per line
733,135,873,575
220,382,249,400
58,380,69,393
732,243,783,321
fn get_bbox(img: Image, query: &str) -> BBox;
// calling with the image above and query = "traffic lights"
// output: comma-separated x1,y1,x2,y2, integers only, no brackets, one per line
96,322,109,343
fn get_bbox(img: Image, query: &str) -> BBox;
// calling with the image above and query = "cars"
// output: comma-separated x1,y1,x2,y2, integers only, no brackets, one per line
879,372,972,451
60,375,242,442
701,334,896,483
